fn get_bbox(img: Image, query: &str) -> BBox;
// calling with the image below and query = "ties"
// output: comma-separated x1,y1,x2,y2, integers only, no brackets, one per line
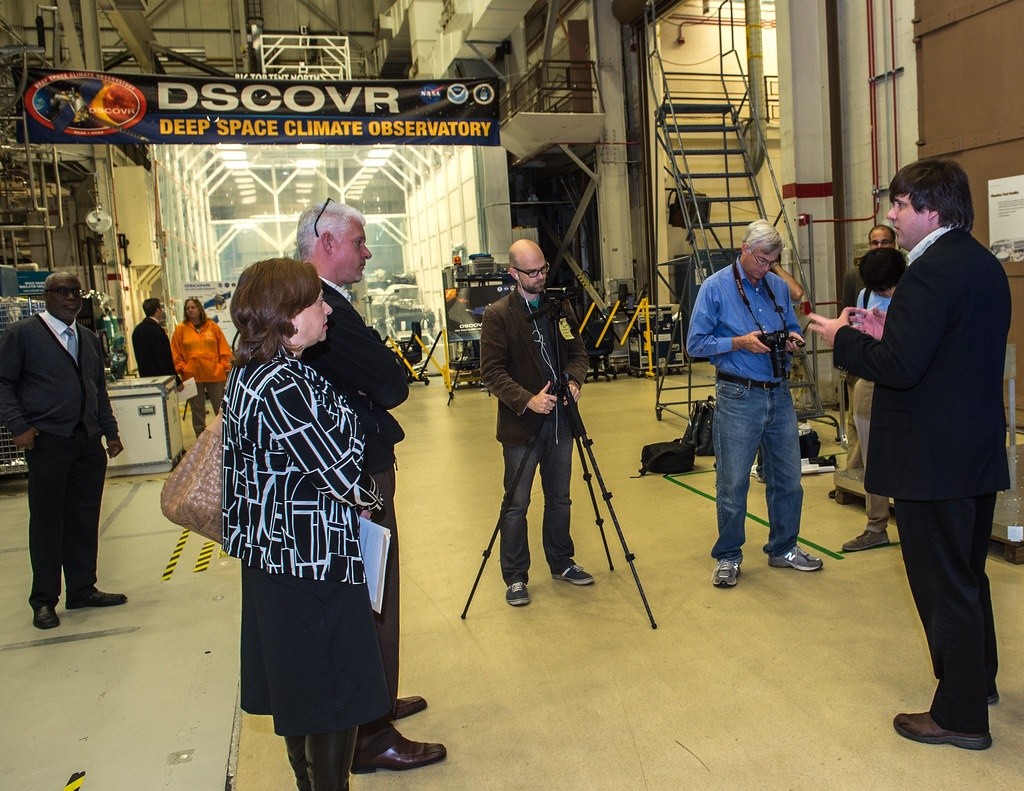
63,327,78,362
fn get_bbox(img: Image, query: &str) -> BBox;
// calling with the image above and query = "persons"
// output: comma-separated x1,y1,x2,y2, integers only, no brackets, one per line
293,203,449,776
222,254,383,791
131,298,184,392
685,218,825,589
886,157,1013,752
0,270,128,630
170,297,233,438
841,223,908,551
479,239,596,607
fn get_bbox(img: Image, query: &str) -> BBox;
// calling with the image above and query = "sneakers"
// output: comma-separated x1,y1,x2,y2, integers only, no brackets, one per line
828,488,836,500
769,545,824,572
551,560,594,585
711,558,743,588
842,530,890,552
505,581,530,607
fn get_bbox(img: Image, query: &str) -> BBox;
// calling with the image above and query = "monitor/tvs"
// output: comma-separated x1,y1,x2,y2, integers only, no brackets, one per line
669,195,711,228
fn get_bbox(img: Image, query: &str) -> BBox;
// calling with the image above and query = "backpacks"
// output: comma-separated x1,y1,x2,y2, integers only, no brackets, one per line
682,400,716,456
630,437,696,480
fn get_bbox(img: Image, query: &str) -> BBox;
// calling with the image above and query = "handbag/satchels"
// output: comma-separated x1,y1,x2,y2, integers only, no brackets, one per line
799,419,821,458
159,411,225,548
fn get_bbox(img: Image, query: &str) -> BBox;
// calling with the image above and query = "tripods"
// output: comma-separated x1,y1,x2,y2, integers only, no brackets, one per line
461,301,658,629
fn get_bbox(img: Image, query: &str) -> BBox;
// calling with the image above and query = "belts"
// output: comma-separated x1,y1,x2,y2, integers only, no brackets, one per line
718,373,781,389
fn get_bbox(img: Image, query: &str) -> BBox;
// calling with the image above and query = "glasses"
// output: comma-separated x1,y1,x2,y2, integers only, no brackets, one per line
511,261,550,277
312,197,336,238
44,286,86,299
750,245,780,268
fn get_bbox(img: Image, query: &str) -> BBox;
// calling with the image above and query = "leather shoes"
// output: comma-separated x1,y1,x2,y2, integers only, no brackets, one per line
389,694,428,719
893,711,992,750
987,691,999,704
353,738,447,777
34,606,61,629
65,591,128,609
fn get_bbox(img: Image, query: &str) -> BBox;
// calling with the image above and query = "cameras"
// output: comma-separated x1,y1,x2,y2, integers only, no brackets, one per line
758,329,789,379
546,286,578,301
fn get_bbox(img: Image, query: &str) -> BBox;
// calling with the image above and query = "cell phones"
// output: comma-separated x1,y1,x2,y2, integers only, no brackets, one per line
788,336,806,347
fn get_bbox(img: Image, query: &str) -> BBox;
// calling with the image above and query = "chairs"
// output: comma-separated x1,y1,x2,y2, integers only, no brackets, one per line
401,321,423,383
584,320,617,383
